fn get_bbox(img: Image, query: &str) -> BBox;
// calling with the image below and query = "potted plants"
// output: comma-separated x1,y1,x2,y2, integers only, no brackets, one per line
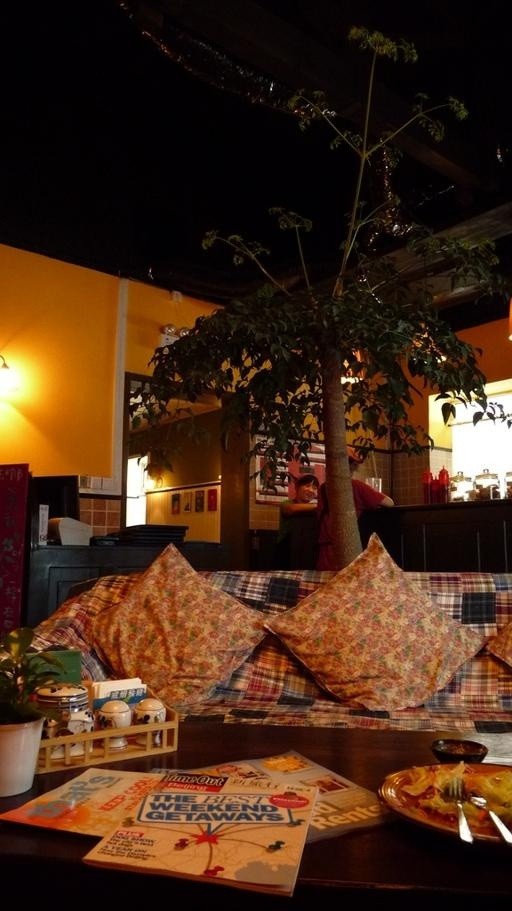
0,627,84,796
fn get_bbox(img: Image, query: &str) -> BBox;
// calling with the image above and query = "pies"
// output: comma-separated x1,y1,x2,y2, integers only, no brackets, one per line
420,766,511,820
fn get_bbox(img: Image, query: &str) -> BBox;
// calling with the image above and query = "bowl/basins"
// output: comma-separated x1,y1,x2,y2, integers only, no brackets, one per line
431,738,489,764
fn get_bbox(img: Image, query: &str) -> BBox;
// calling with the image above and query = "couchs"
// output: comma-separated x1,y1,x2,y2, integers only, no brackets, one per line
32,570,512,724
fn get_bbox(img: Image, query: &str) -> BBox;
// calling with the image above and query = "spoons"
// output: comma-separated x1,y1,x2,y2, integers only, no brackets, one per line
467,796,512,845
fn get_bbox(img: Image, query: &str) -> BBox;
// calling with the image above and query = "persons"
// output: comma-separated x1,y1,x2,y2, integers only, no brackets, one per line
316,445,396,571
277,472,321,571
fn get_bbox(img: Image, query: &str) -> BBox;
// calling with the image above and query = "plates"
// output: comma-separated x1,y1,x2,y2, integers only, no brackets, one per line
118,523,189,548
377,763,512,846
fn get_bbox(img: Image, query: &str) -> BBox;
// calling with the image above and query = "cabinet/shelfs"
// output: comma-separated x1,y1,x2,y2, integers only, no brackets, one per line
26,547,225,626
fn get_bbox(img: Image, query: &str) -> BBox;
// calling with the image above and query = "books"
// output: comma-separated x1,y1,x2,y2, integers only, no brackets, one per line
21,649,83,705
197,752,396,848
83,772,320,897
1,768,173,838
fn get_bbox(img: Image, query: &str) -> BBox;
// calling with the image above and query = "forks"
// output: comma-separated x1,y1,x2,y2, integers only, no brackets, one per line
447,775,475,844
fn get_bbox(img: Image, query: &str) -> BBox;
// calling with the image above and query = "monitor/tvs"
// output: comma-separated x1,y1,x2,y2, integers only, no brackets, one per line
34,474,80,522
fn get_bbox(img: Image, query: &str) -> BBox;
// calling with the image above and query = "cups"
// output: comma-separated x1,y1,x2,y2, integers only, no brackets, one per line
365,477,382,494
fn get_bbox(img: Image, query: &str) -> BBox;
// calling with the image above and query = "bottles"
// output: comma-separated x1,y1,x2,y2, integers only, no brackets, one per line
421,465,512,507
134,698,166,746
35,682,93,762
96,701,132,750
